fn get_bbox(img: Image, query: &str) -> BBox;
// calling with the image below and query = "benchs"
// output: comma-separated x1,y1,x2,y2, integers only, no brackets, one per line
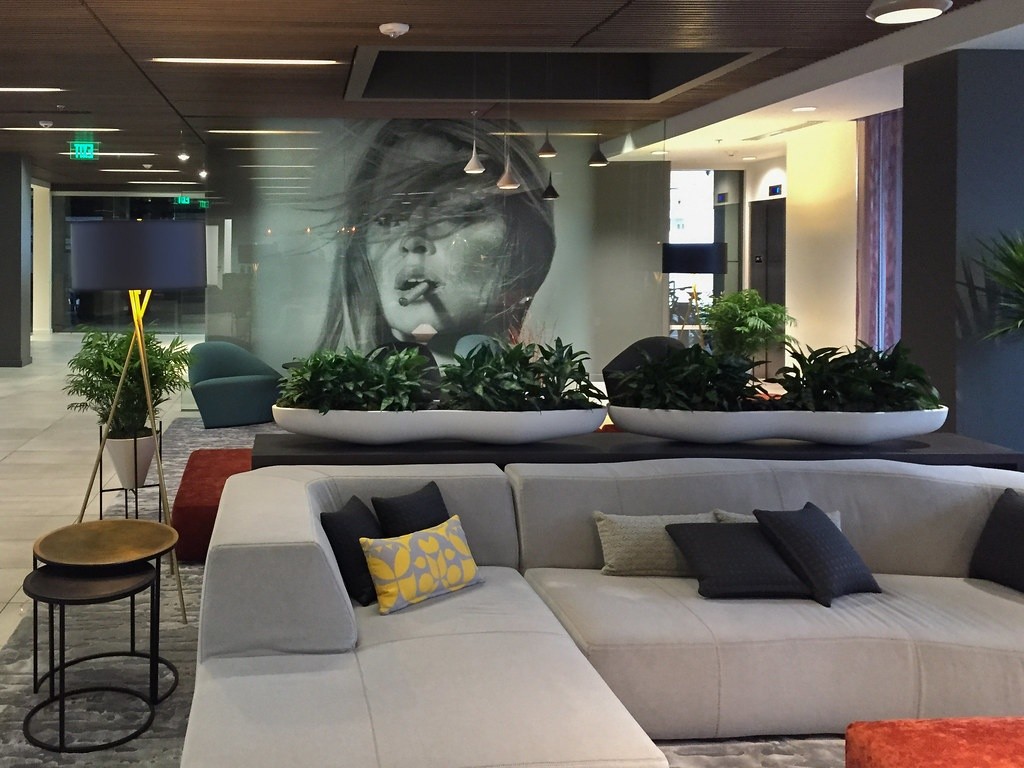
171,447,254,564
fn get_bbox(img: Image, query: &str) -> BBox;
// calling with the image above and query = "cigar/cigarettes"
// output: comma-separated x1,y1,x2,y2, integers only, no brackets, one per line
399,280,434,307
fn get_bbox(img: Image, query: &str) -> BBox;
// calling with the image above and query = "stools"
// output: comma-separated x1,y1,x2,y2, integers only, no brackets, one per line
22,519,179,752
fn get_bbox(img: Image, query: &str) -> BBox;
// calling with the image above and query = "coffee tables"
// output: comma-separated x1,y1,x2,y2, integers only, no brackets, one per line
845,716,1024,768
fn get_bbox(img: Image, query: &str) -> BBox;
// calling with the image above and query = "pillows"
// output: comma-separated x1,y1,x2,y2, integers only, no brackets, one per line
320,481,485,616
591,500,883,608
967,487,1024,596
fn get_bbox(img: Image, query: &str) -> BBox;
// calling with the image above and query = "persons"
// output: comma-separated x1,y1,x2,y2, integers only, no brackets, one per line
287,115,557,357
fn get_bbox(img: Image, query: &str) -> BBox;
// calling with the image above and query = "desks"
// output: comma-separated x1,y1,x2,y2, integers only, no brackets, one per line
251,432,1024,495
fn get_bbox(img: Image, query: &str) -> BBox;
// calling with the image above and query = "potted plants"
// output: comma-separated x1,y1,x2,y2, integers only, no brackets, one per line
270,333,607,447
61,321,192,488
607,288,951,447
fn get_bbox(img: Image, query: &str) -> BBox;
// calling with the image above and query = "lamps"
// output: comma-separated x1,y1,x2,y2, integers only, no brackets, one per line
536,127,558,157
542,171,560,201
864,0,953,24
464,109,485,174
588,134,608,169
497,108,520,190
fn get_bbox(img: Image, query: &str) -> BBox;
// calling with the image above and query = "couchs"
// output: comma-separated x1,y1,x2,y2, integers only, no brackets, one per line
504,458,1024,740
179,461,670,768
188,341,288,429
